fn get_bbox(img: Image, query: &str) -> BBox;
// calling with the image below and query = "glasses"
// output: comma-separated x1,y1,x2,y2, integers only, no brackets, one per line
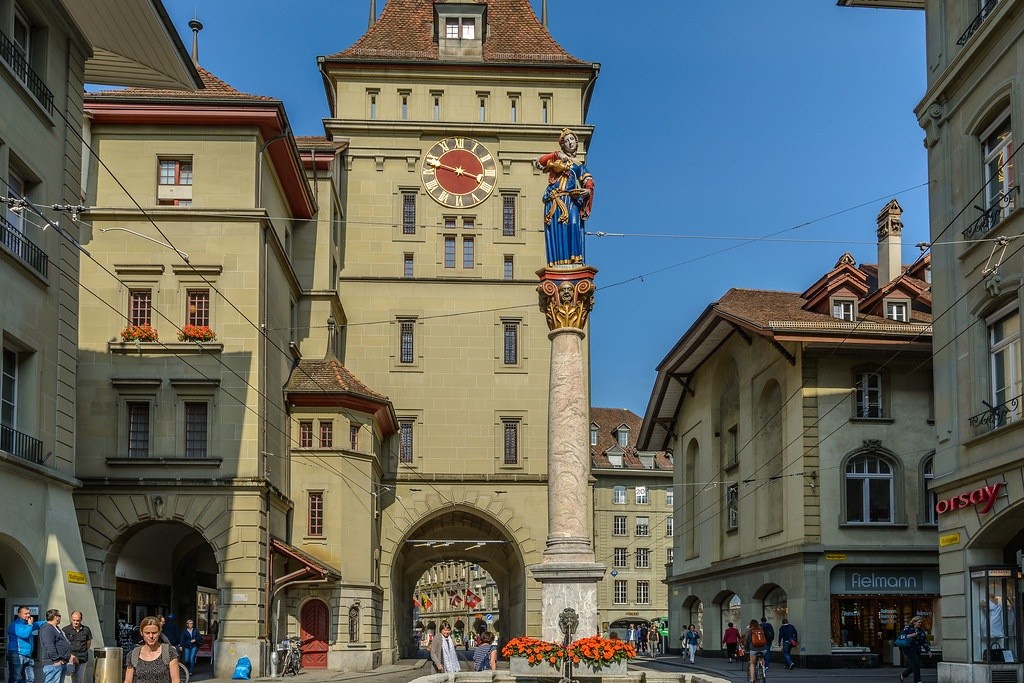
55,615,62,617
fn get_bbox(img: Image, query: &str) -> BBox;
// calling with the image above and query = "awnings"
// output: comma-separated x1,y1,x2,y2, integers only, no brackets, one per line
272,538,340,585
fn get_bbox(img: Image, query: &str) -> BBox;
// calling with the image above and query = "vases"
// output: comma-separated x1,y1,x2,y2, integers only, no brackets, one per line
509,655,566,678
571,657,628,678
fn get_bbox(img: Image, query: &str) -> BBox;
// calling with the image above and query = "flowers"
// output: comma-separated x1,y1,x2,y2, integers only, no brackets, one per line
501,636,565,670
120,320,160,342
176,324,218,343
566,632,637,669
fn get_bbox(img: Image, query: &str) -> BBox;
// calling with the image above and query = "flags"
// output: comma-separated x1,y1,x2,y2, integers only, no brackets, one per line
420,593,433,610
413,597,421,606
464,590,482,608
450,590,462,606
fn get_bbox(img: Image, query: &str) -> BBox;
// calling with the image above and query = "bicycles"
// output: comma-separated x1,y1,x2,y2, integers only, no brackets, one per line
744,649,768,683
280,637,302,677
178,661,189,683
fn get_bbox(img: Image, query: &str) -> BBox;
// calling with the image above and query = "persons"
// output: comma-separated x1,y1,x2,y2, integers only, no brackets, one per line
539,128,594,265
179,619,202,675
39,609,80,683
897,616,933,683
211,621,219,635
722,622,740,663
124,617,181,683
640,624,648,652
626,624,638,649
473,632,498,671
160,614,180,646
647,626,659,657
62,611,92,683
681,625,689,660
431,622,461,674
429,634,433,640
981,592,1003,637
7,605,47,683
635,625,641,653
744,617,798,683
686,624,700,664
413,633,420,650
465,631,481,650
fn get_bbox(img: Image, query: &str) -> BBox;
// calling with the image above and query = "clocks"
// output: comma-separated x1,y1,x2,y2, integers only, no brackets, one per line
420,136,499,209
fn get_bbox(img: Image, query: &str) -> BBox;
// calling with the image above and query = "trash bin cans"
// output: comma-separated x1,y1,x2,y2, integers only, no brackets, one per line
270,651,282,676
93,647,123,683
660,636,667,655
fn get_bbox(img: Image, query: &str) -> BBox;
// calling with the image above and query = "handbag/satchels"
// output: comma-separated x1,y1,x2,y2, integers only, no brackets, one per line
739,642,745,656
790,639,797,647
477,645,498,672
679,636,684,640
983,642,1006,663
893,634,914,647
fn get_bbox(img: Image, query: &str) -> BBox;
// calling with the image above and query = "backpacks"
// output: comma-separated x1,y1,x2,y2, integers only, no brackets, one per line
764,627,774,642
751,629,767,647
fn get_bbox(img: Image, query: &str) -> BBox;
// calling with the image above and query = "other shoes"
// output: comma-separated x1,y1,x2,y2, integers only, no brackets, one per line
629,647,663,659
788,663,794,670
680,653,701,664
189,673,192,677
728,661,732,663
899,673,904,682
784,665,789,669
